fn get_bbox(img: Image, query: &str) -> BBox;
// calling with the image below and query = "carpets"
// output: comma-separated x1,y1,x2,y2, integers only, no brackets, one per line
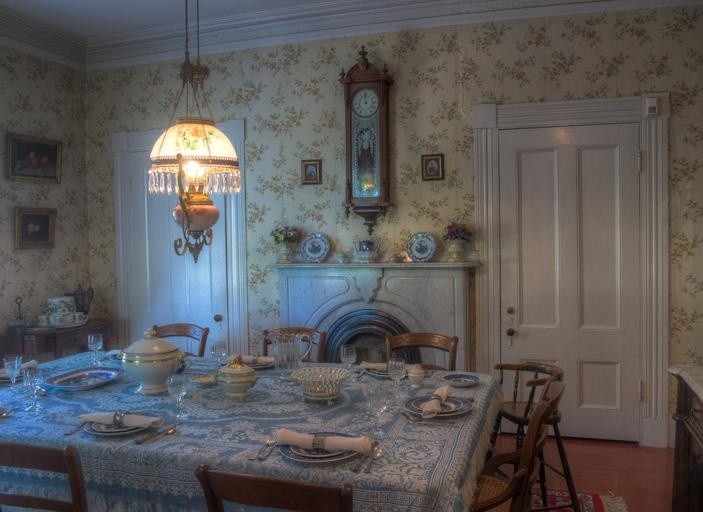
528,485,629,512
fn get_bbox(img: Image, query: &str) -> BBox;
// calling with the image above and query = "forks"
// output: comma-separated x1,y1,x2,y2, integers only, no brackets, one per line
399,410,425,427
353,369,365,382
248,433,279,460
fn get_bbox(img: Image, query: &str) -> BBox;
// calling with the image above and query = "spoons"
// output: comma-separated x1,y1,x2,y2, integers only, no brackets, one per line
350,441,383,475
1,409,16,418
134,423,175,444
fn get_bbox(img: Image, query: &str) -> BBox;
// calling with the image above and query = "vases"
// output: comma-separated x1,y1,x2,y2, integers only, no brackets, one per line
276,242,296,264
446,238,467,263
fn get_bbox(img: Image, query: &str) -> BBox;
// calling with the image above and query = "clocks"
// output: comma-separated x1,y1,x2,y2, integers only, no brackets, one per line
337,44,394,237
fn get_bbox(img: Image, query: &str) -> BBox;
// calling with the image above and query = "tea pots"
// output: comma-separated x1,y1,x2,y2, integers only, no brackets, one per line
352,240,380,264
266,333,311,378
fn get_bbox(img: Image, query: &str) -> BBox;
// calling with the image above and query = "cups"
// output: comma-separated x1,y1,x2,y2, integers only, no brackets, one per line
366,382,390,435
21,296,88,328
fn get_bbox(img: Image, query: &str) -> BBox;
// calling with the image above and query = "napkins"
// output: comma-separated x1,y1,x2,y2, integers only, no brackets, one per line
76,413,162,429
422,386,452,419
103,350,122,360
358,361,421,373
0,361,39,377
272,428,372,454
228,354,274,365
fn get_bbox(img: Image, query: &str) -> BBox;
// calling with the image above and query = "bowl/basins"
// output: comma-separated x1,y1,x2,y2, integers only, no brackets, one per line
335,254,350,264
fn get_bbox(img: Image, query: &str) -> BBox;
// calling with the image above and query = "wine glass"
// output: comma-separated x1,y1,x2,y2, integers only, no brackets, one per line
167,375,191,423
3,356,44,413
388,359,405,389
340,345,355,369
87,334,104,367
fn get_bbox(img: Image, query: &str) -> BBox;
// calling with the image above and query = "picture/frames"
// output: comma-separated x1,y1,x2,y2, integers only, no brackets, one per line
420,153,445,181
3,131,64,186
12,205,59,251
299,159,322,185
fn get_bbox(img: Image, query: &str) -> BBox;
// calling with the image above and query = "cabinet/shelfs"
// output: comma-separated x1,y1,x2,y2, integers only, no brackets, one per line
665,360,702,511
0,317,115,360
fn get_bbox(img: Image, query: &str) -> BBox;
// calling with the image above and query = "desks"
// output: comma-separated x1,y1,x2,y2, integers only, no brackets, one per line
2,350,501,512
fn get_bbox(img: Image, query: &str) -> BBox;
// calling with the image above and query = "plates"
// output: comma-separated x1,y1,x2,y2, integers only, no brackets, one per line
189,375,217,385
290,433,344,458
405,232,435,263
279,431,364,463
46,367,121,391
82,411,157,436
442,373,480,387
89,423,136,433
225,354,273,369
362,365,405,381
0,368,24,383
300,232,330,263
404,397,472,418
410,397,463,412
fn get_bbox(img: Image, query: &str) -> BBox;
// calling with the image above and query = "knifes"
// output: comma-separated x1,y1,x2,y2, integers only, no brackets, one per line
64,422,84,436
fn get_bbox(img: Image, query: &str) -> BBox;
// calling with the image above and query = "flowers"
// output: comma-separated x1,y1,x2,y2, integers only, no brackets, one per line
442,220,475,242
269,223,304,252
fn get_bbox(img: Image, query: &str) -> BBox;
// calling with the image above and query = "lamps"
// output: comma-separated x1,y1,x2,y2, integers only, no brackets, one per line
145,1,243,266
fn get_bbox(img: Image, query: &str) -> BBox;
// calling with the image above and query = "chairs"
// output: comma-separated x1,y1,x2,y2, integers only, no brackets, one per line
261,326,325,364
0,441,88,511
387,332,459,371
195,464,354,512
476,361,578,510
147,324,209,357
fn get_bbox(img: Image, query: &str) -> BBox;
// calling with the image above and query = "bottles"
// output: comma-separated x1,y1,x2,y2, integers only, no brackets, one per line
116,325,187,395
407,364,424,388
216,356,259,397
